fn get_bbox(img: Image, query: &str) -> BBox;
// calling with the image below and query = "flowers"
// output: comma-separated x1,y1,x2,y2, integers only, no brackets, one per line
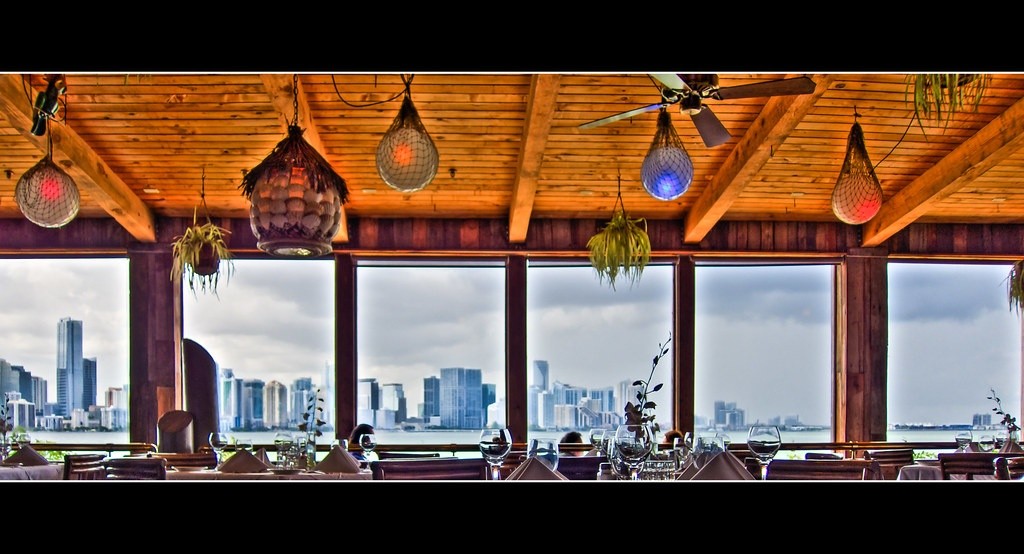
986,387,1021,441
624,330,671,446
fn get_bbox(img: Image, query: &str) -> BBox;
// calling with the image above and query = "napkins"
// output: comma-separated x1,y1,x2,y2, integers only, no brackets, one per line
676,448,716,480
998,437,1023,453
218,449,270,472
688,450,757,480
503,457,570,481
3,445,50,465
305,444,362,473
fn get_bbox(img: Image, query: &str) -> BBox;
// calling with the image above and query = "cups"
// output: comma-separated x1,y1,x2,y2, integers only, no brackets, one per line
601,431,644,480
527,438,560,472
331,439,348,452
997,430,1019,446
699,437,724,453
17,433,31,448
638,461,675,480
235,438,253,455
978,435,995,452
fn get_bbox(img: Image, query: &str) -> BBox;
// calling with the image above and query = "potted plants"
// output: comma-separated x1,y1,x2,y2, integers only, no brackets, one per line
169,201,236,302
585,213,651,290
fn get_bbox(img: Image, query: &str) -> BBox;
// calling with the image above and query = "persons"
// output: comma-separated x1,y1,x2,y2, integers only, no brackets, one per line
556,432,584,457
346,424,378,462
662,432,684,444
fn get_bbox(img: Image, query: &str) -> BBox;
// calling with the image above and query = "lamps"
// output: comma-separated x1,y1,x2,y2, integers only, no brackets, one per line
238,75,349,257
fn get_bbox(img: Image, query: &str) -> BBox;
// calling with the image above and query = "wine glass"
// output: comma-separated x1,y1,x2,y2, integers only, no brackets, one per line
360,434,376,473
615,424,653,480
590,429,606,456
746,426,781,480
209,433,228,471
274,432,293,467
955,430,971,452
673,438,692,468
480,429,512,480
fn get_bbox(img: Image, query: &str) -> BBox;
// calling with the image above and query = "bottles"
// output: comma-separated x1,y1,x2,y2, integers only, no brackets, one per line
597,464,612,480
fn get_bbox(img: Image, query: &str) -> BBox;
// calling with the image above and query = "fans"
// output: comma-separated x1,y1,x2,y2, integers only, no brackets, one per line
576,73,818,152
